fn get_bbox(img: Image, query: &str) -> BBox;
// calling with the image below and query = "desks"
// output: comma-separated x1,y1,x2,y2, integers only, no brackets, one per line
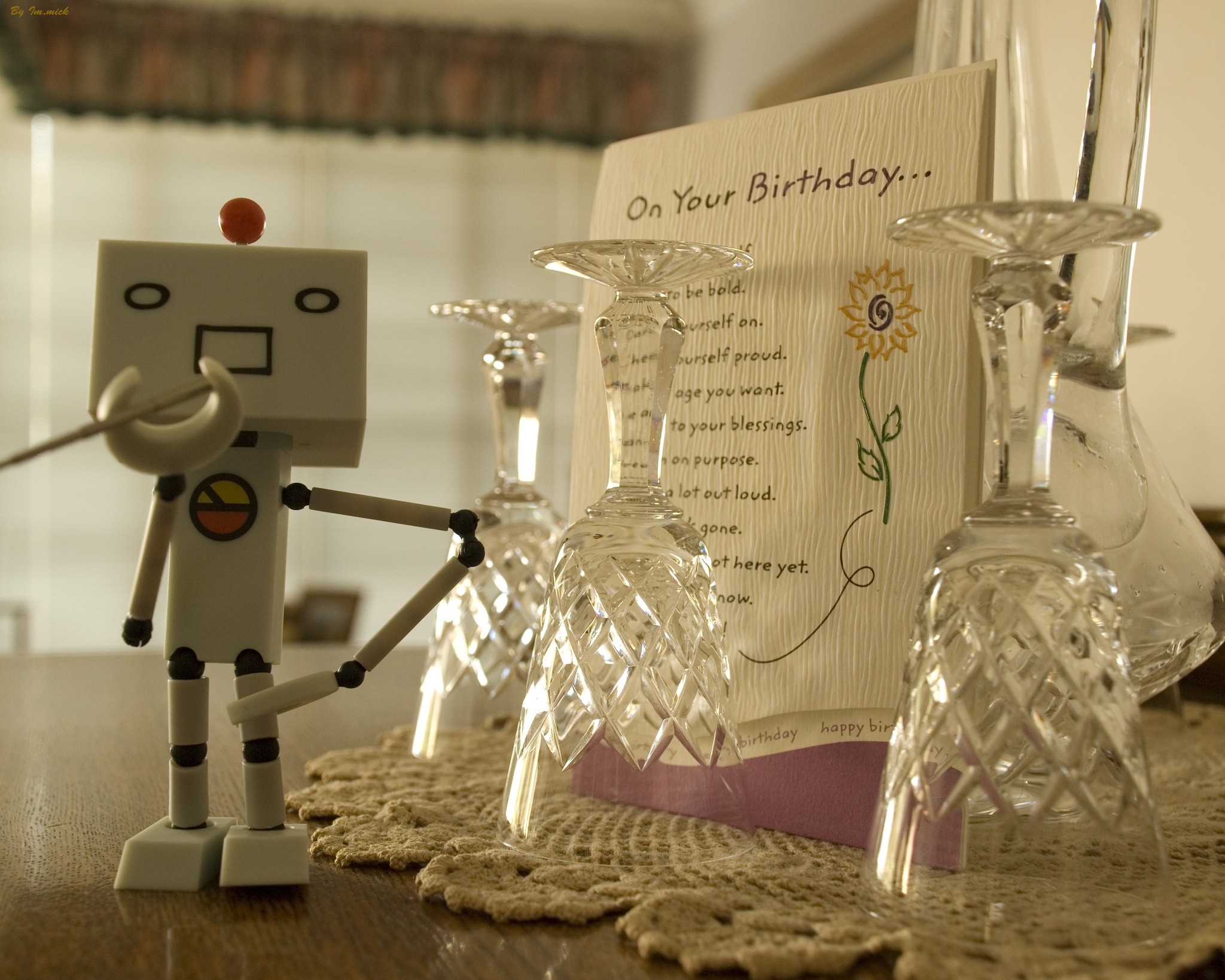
0,647,1223,980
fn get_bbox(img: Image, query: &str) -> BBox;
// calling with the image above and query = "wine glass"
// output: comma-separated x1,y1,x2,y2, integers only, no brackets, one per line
406,296,583,766
856,199,1181,962
494,237,764,870
